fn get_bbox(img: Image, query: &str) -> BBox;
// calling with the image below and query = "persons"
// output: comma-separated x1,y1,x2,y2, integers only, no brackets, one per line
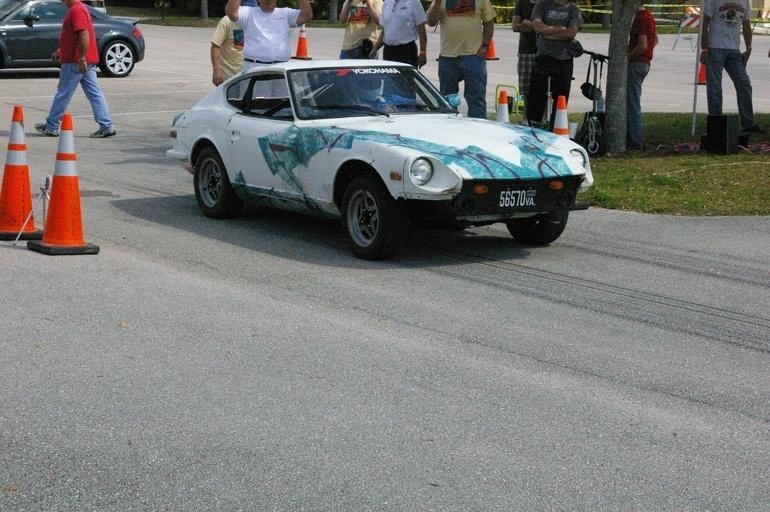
525,1,584,133
427,0,498,118
361,77,417,113
624,6,659,152
699,1,765,134
210,0,259,87
338,0,384,59
512,0,539,111
34,0,117,139
225,0,315,99
369,0,428,98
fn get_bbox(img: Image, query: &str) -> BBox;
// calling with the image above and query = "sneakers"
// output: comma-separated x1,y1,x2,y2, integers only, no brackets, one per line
35,124,59,136
90,128,116,138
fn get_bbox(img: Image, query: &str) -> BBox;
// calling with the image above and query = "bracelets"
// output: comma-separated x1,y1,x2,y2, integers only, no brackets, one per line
746,46,752,49
420,50,426,55
482,44,489,50
701,48,708,51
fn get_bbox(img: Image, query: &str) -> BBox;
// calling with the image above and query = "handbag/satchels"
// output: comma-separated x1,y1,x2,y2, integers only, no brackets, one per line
580,82,602,100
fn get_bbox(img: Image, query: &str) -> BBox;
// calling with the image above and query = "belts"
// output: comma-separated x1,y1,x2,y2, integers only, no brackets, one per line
244,58,280,63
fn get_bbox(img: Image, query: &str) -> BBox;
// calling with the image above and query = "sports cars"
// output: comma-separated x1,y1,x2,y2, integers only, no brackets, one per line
166,59,594,258
0,0,147,79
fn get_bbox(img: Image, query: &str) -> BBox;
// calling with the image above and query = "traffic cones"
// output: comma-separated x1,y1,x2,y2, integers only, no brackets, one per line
485,37,501,60
25,112,104,254
496,91,512,125
550,94,571,140
694,48,707,84
0,106,43,240
292,23,311,60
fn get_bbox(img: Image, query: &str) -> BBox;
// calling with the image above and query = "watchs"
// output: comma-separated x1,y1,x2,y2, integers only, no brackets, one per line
79,57,85,63
